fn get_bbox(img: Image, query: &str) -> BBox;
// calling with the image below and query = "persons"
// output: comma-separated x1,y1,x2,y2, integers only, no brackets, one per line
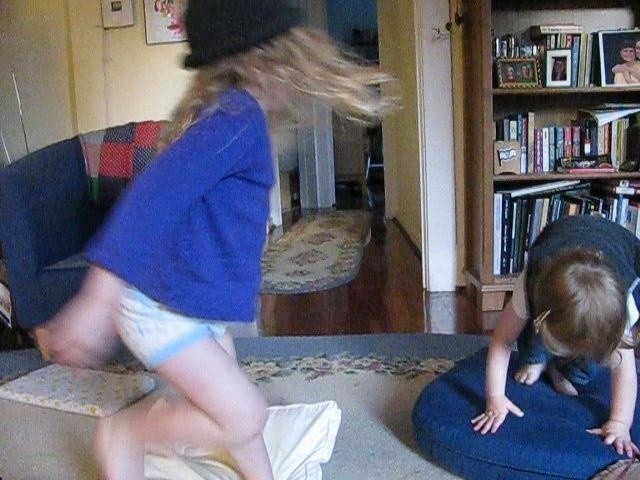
470,215,640,458
46,2,398,480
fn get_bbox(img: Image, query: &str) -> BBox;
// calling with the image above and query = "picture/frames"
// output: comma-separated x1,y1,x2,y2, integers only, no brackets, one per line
598,28,640,87
143,0,190,45
496,47,574,89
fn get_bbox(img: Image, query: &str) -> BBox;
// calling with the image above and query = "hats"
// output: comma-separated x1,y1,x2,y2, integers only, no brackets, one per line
179,1,309,69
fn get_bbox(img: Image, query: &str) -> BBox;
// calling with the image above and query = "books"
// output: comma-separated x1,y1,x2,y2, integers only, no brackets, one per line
491,22,640,277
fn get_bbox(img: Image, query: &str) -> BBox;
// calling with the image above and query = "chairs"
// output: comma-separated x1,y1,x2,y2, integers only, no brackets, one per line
1,120,191,332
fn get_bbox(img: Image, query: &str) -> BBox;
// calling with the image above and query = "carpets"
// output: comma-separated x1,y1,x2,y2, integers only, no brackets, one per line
1,333,522,478
257,207,374,295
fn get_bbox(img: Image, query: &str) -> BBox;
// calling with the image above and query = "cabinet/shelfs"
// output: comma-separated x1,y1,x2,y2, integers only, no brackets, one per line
461,0,640,313
